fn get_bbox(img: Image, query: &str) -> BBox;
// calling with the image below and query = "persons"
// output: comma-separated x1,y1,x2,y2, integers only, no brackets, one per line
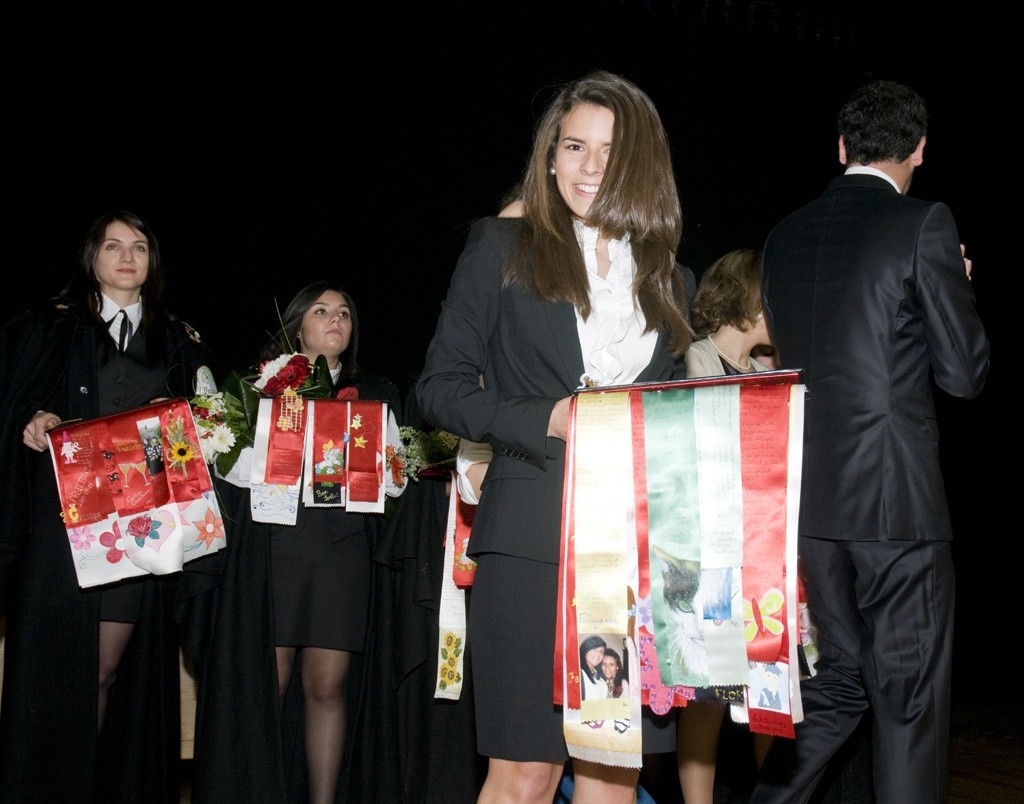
12,208,208,804
680,243,779,395
424,70,708,804
579,635,629,700
220,280,396,804
762,76,996,804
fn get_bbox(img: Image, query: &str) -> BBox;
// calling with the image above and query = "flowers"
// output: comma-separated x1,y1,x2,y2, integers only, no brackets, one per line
406,424,468,478
250,350,330,400
144,374,237,473
